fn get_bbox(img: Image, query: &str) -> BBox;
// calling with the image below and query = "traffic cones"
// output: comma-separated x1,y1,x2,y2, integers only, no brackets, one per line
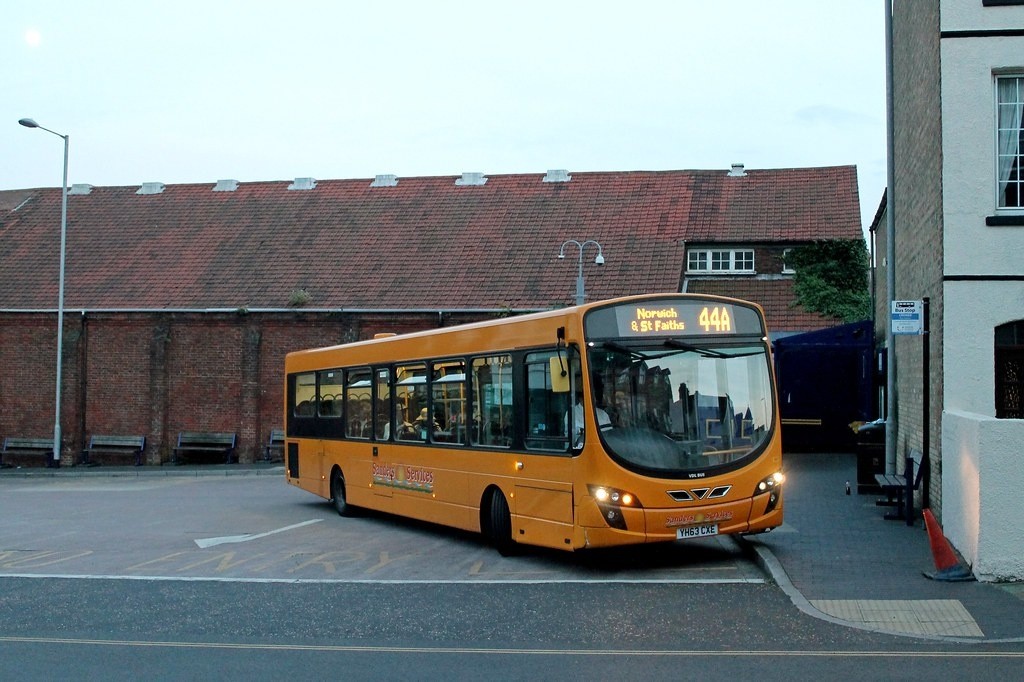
921,508,977,580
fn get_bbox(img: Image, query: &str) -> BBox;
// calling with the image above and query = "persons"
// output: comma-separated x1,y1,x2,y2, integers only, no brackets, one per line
383,404,414,440
416,408,441,434
565,387,613,449
351,398,372,437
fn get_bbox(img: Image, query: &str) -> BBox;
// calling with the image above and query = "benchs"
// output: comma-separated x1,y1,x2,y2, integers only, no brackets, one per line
0,437,59,468
82,434,146,466
265,429,284,460
172,432,237,464
875,447,923,527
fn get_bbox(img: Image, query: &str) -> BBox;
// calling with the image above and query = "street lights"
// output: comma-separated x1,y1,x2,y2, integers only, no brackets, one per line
558,239,605,304
17,117,70,466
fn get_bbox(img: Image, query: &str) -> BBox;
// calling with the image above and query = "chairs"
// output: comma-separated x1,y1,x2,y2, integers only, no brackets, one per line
297,393,503,446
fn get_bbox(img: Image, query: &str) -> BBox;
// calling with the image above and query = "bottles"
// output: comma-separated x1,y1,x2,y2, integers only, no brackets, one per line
846,480,850,495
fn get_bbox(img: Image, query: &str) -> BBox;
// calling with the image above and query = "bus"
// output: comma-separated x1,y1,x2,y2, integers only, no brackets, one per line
282,291,784,559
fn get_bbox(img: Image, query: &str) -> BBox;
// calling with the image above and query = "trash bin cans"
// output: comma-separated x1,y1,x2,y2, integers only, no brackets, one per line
855,420,887,494
